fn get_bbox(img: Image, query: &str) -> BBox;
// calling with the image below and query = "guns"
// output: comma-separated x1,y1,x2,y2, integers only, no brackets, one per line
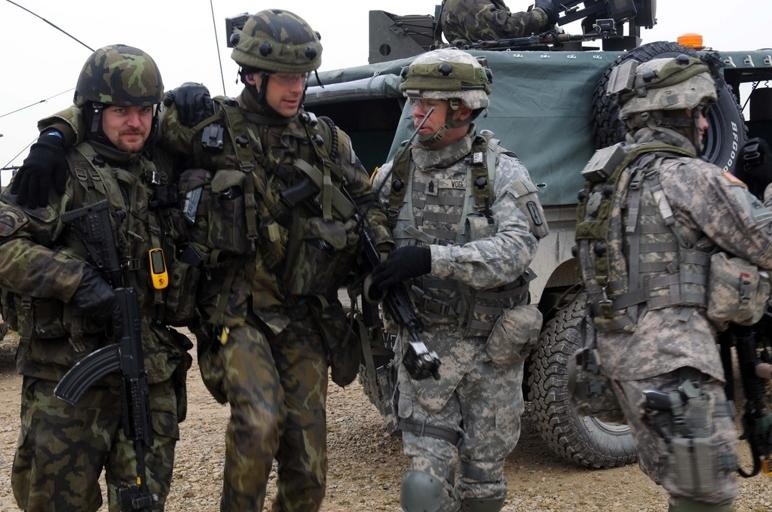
281,164,356,223
429,25,623,51
354,214,440,380
720,138,772,478
54,200,157,512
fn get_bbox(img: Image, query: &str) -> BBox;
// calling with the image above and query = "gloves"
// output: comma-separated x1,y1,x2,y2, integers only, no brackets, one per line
74,262,117,326
165,83,214,128
12,134,73,211
534,1,560,25
371,244,431,292
148,182,180,208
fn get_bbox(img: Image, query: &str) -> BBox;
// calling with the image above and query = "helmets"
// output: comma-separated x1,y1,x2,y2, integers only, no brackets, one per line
71,43,165,107
400,48,492,112
617,53,718,120
231,7,322,76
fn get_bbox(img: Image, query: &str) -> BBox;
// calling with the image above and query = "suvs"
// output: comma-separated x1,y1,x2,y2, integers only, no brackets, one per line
290,44,772,470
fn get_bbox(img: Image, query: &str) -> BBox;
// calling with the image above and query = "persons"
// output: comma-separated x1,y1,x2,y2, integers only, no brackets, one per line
573,54,770,511
0,44,187,511
439,0,559,46
12,8,397,512
362,46,549,512
